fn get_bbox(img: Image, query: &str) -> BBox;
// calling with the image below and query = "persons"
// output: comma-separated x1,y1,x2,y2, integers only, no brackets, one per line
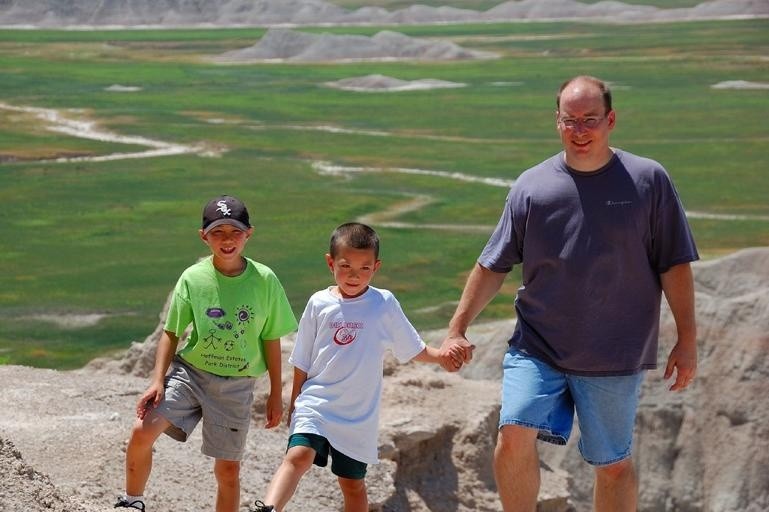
246,222,474,512
437,76,702,512
110,194,300,512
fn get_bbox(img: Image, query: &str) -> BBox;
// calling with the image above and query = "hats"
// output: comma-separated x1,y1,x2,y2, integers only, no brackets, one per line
203,196,251,234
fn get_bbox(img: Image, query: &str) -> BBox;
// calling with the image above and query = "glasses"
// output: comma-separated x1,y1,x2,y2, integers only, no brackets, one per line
561,116,605,131
